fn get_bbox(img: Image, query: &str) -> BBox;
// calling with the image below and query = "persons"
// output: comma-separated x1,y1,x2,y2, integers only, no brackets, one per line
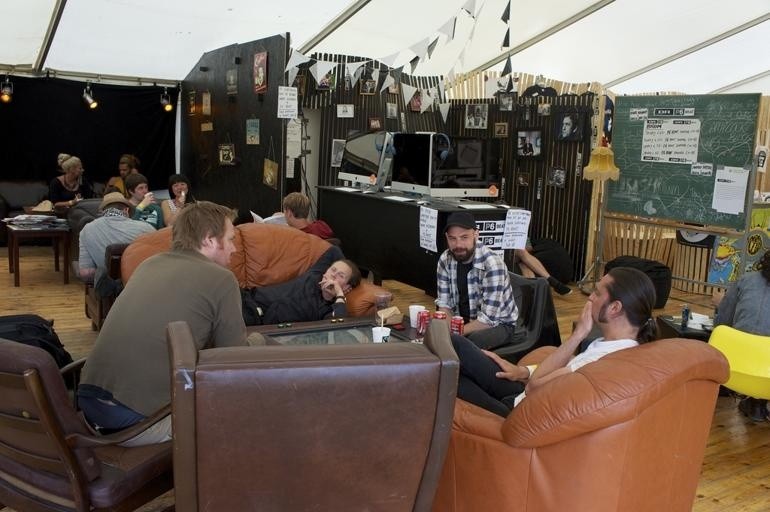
47,152,199,295
238,245,361,325
435,209,519,350
523,137,534,156
275,192,335,238
450,266,660,420
496,199,572,296
710,249,770,420
77,199,263,446
557,113,581,141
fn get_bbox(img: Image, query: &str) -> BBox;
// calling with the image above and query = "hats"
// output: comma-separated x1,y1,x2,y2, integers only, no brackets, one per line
98,191,136,215
442,210,478,235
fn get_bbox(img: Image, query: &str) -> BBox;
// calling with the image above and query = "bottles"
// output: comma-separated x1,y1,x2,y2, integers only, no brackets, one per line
681,304,689,329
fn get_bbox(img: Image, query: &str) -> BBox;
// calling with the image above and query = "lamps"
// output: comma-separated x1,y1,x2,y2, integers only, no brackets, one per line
0,62,180,114
581,145,621,298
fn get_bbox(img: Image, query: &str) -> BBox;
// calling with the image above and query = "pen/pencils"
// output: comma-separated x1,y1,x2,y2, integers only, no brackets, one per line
684,223,704,227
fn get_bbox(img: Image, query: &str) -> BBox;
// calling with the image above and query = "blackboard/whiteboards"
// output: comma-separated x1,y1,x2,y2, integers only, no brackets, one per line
603,92,763,236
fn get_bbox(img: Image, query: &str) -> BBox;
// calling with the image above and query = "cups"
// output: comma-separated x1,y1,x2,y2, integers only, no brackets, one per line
372,326,391,344
409,305,425,329
145,192,155,206
375,291,393,326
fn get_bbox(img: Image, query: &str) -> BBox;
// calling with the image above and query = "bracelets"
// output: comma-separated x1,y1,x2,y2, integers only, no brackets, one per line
334,295,348,300
526,363,538,379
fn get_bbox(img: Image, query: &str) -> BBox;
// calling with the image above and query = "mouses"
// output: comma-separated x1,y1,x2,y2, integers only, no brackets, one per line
417,200,431,205
497,204,510,208
363,191,376,194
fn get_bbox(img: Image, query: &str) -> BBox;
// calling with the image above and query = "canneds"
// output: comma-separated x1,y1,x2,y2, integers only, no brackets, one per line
416,310,430,339
451,316,465,336
433,311,447,319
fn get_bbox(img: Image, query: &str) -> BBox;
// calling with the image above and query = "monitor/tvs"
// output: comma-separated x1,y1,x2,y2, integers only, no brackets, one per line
428,133,500,201
391,131,435,197
337,129,389,185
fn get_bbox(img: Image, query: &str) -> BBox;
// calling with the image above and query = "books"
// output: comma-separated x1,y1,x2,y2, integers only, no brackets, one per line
250,210,290,225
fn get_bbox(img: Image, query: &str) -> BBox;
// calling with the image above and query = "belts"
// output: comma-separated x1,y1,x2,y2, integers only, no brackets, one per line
246,287,266,326
81,409,141,436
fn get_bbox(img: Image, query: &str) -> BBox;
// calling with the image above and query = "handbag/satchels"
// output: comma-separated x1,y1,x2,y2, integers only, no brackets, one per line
603,254,672,309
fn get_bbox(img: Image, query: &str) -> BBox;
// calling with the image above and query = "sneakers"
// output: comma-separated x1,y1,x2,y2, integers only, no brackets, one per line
738,393,768,425
553,282,573,297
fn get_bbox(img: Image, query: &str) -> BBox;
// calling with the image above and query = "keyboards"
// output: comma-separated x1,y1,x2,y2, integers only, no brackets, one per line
384,196,416,202
458,204,497,210
335,187,362,192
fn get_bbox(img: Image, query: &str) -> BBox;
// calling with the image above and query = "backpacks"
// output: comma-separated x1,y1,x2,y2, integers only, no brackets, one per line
0,313,84,392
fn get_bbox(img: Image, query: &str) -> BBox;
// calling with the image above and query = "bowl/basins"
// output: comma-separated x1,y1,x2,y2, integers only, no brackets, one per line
700,319,713,330
